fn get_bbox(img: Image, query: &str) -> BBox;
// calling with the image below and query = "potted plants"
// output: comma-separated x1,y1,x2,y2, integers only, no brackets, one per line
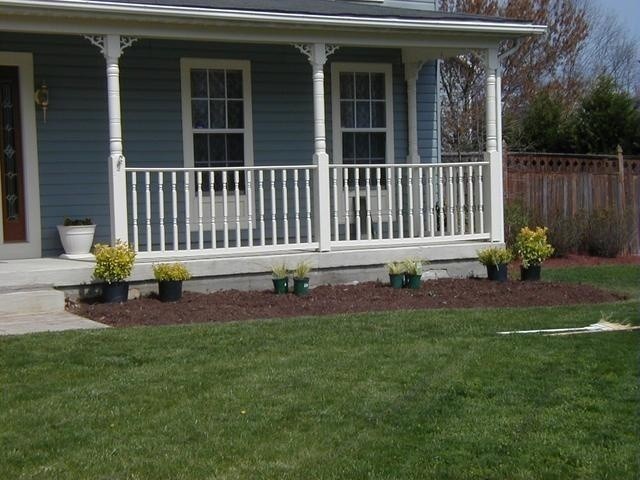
387,258,423,289
154,263,190,302
56,214,97,254
92,240,137,302
479,225,557,281
270,261,311,296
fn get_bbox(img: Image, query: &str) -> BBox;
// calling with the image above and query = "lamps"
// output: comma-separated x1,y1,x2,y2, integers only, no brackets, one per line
35,78,51,125
